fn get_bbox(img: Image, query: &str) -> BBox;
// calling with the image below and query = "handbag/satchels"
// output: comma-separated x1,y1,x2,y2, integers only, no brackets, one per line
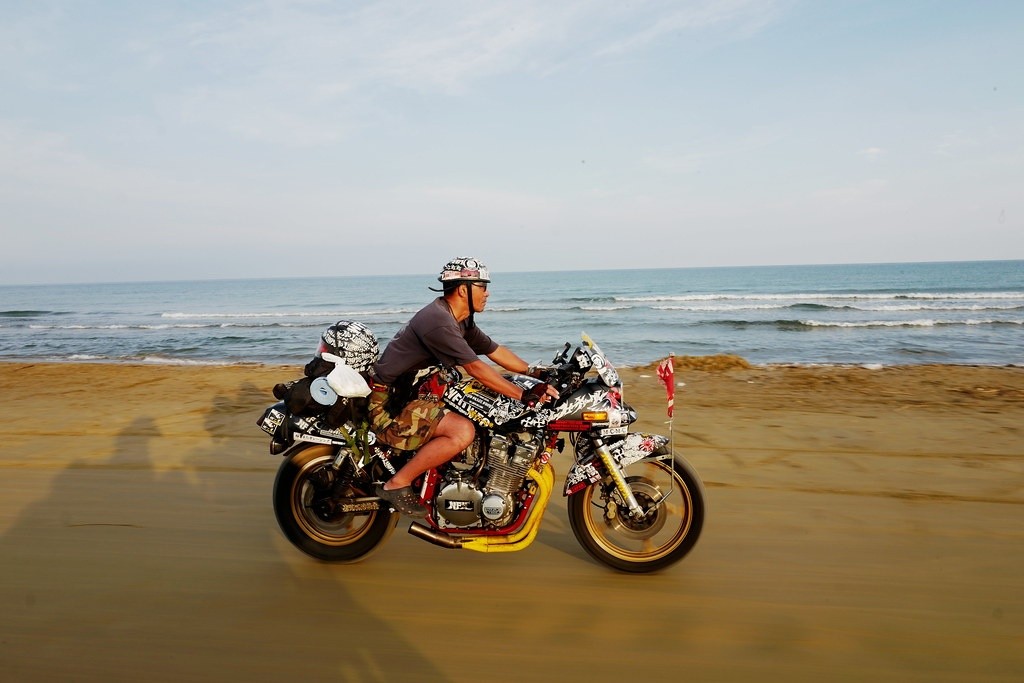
282,318,380,426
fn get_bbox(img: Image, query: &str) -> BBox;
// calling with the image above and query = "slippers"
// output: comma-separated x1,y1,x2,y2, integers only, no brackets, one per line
375,485,427,518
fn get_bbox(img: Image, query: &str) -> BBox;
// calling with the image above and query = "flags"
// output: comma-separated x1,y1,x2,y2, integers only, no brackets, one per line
657,359,674,416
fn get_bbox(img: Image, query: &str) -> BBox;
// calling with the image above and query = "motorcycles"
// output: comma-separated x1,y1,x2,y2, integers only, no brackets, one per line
256,330,705,576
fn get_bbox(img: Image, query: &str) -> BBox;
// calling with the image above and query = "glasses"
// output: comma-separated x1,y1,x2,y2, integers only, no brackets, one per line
472,282,486,291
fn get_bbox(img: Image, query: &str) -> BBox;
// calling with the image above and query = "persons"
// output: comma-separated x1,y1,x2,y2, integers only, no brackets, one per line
365,257,560,520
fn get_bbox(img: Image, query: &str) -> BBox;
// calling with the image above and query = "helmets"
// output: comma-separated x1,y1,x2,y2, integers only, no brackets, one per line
438,256,491,282
